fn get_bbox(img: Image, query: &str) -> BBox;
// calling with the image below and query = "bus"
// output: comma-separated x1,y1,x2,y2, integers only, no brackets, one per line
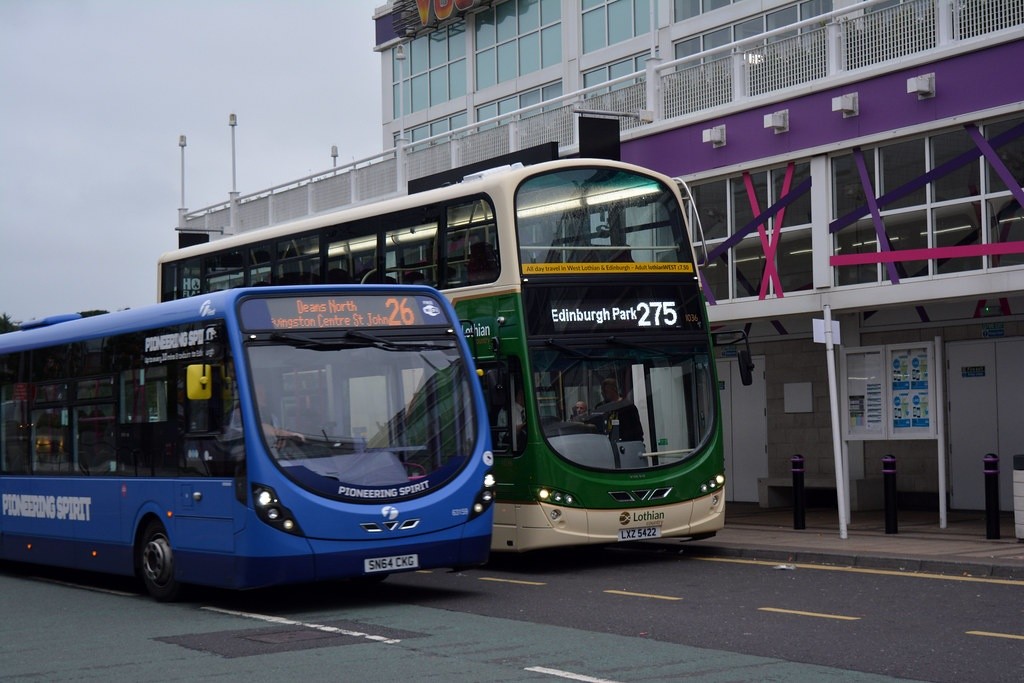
0,285,503,607
156,158,755,566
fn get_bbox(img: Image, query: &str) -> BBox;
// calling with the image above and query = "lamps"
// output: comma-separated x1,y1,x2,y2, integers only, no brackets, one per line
764,109,789,136
831,91,859,119
702,124,727,148
907,72,936,100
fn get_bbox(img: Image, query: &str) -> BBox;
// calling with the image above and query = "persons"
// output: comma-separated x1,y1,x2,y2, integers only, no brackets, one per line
219,379,305,461
573,378,644,442
497,379,527,449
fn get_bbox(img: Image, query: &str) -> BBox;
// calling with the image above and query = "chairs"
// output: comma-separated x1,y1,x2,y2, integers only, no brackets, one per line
189,267,429,295
470,241,500,281
